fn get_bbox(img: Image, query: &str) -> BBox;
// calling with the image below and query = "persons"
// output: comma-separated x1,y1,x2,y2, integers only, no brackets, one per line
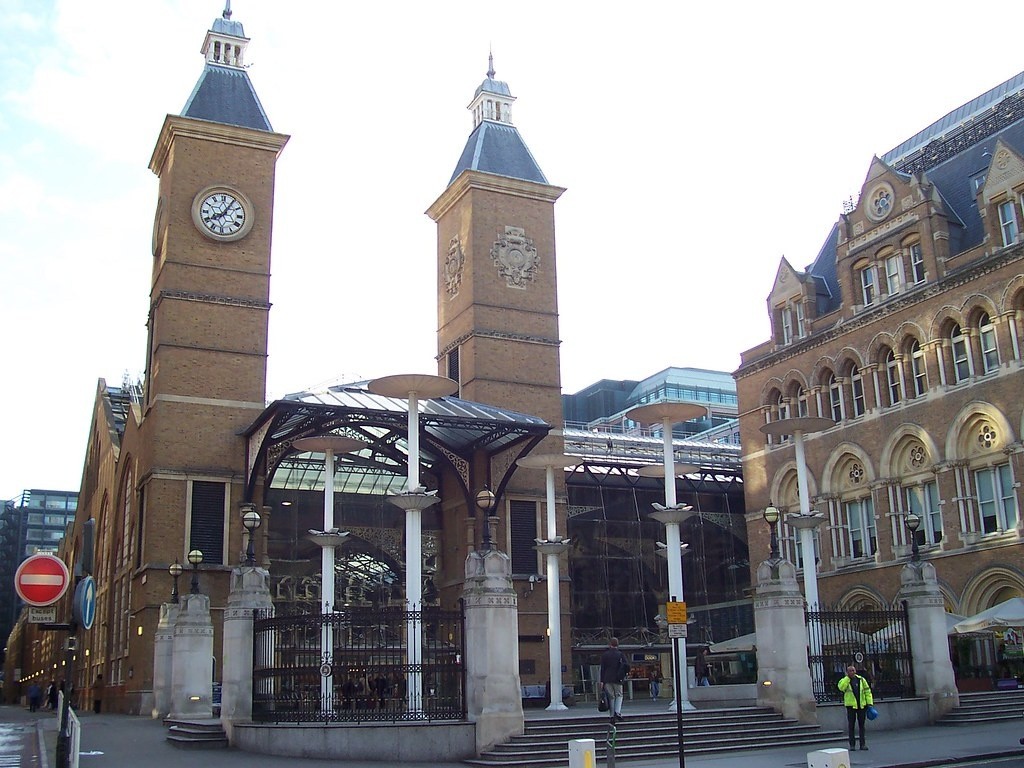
648,665,664,702
694,647,710,687
28,674,104,714
600,638,630,723
838,666,873,750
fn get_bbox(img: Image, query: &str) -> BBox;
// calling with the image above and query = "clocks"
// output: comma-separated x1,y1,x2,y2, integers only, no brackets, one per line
190,184,255,245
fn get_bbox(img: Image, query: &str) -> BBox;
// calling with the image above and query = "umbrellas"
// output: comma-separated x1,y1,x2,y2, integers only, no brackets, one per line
873,610,968,644
806,622,872,649
709,632,758,653
954,597,1024,633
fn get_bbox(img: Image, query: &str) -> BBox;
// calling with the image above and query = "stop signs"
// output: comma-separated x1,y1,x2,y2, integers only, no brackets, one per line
14,554,70,607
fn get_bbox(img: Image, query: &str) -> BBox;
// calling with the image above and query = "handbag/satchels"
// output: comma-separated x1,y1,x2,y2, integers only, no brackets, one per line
618,652,631,682
598,686,610,712
867,706,878,721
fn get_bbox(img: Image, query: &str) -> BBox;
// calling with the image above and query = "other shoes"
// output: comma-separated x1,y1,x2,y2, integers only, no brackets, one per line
608,716,613,724
850,746,855,751
861,745,868,750
614,712,625,722
